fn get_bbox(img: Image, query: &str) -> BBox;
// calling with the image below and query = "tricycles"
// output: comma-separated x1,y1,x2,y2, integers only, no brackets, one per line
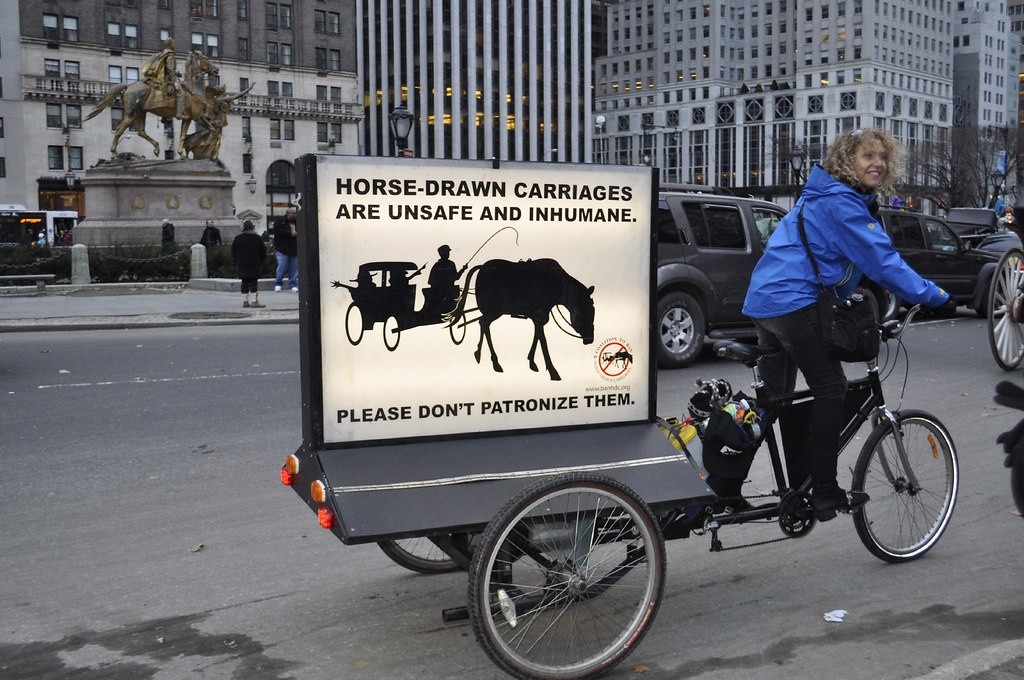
274,150,967,680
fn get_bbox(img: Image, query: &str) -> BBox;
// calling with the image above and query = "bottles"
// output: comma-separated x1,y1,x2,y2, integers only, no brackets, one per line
699,399,750,436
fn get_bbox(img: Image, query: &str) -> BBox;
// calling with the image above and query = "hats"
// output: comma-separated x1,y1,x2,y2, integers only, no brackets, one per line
243,221,255,232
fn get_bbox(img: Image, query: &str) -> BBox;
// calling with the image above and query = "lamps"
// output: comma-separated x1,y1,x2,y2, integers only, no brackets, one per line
245,174,257,195
64,168,76,190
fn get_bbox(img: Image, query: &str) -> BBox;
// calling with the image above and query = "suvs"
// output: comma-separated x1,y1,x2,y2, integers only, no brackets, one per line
655,180,793,371
873,203,1024,327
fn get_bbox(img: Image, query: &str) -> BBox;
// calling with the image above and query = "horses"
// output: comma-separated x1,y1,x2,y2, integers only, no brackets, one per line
79,50,220,161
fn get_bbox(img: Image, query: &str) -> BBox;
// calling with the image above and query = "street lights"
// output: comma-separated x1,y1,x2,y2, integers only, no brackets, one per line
788,145,805,205
386,100,415,156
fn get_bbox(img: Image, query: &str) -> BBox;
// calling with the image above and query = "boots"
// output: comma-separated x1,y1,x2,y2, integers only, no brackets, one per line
242,293,250,306
706,444,755,511
251,293,265,307
809,454,869,522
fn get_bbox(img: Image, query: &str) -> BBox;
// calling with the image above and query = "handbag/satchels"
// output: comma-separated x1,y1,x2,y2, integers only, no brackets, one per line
816,287,882,362
702,391,766,478
689,378,733,421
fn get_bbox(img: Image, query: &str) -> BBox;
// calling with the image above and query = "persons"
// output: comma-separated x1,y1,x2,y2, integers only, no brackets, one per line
232,220,267,308
273,209,299,292
37,233,45,245
161,218,175,245
183,81,256,161
162,37,190,120
200,219,222,246
23,229,33,244
705,128,956,531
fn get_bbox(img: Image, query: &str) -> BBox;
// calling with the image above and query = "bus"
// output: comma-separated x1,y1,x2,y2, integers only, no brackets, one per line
0,209,80,265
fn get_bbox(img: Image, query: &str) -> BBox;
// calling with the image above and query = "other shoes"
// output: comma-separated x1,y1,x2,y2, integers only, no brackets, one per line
291,286,299,292
275,286,281,291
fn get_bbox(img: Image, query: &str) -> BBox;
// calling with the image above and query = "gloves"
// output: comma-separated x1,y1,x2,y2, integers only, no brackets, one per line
923,293,957,320
